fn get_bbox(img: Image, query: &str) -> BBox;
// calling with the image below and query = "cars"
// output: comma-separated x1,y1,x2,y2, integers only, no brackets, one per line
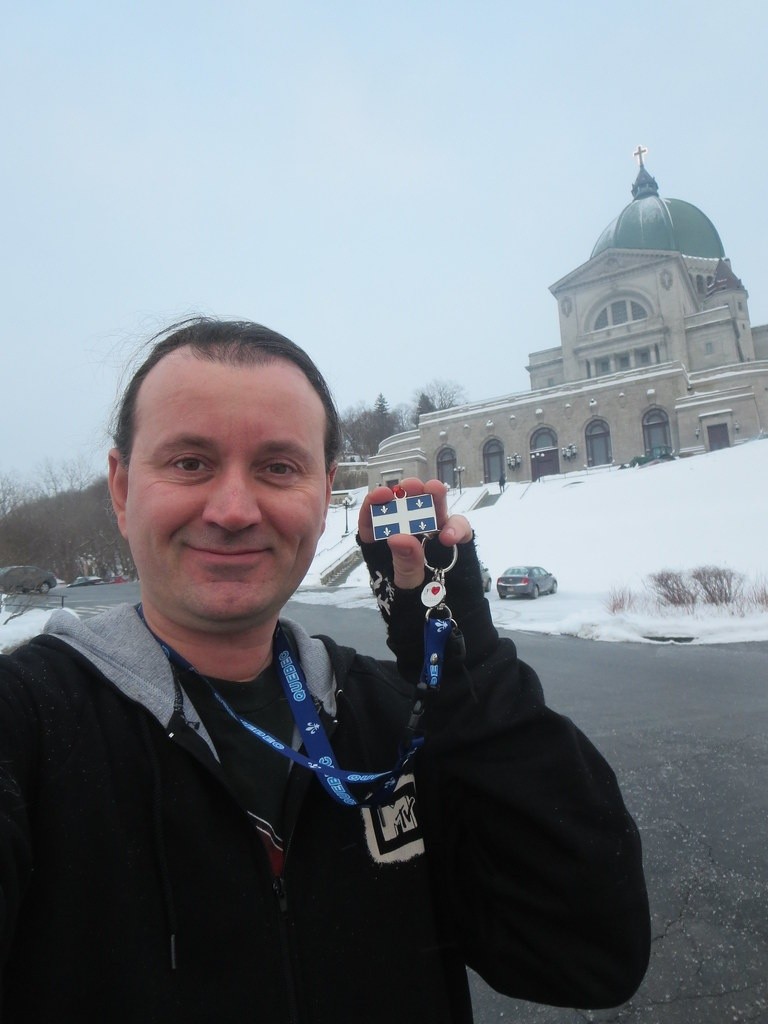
478,561,491,593
66,576,103,588
0,565,58,594
497,566,557,599
109,576,126,584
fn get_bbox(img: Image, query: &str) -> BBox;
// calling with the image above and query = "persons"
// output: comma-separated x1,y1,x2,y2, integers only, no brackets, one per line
0,315,654,1024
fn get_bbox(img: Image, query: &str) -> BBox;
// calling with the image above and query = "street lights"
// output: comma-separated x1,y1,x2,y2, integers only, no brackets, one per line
454,466,465,495
342,497,350,533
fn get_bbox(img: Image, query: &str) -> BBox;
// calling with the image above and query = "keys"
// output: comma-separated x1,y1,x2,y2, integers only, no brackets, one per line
445,627,481,707
